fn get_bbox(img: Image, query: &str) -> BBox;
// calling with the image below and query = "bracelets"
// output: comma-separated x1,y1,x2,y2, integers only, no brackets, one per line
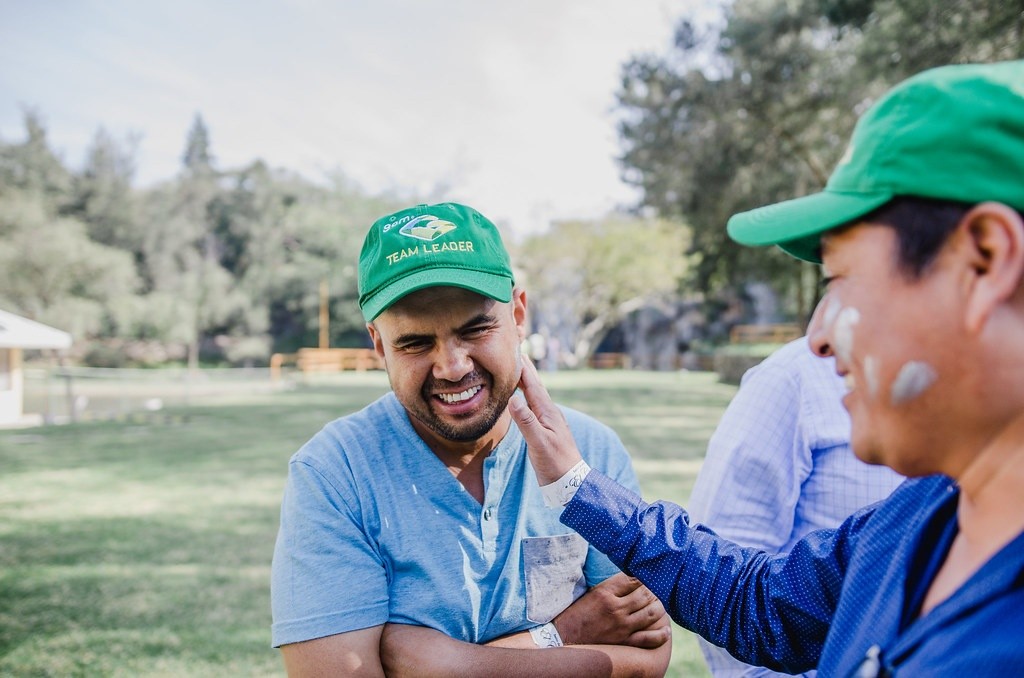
528,622,564,648
537,459,592,510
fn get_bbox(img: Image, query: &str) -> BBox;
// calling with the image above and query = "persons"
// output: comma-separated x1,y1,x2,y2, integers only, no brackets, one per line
509,63,1024,676
269,202,674,678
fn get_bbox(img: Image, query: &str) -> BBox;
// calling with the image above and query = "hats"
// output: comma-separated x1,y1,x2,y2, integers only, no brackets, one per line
725,60,1024,244
357,202,516,323
